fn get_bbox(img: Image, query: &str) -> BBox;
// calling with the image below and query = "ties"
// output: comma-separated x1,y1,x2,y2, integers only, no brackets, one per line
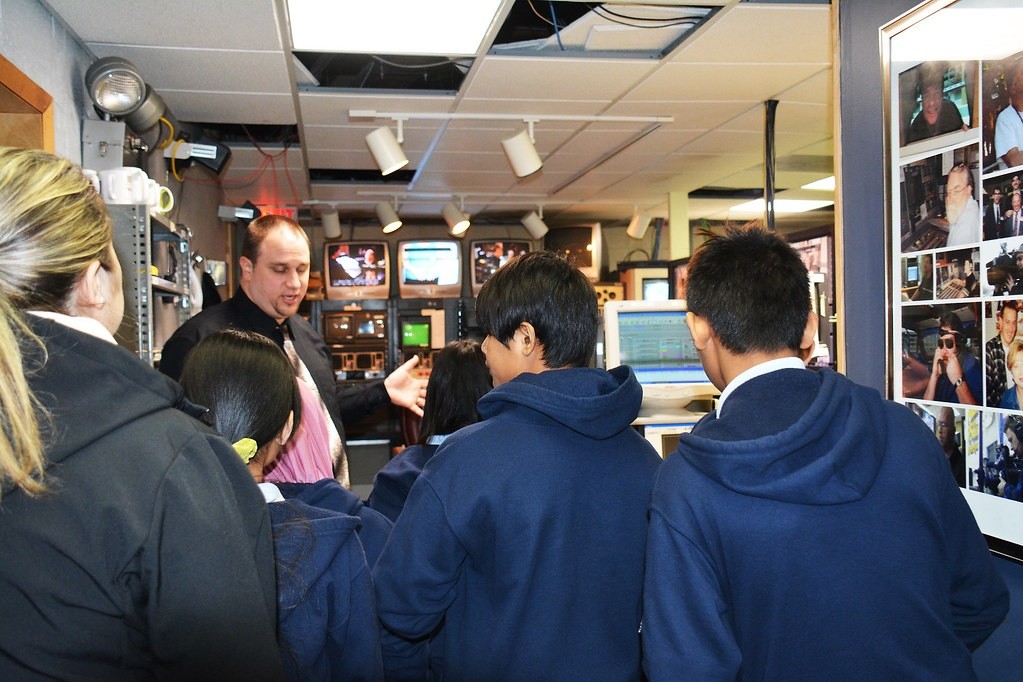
277,324,351,496
997,205,999,223
1013,212,1019,237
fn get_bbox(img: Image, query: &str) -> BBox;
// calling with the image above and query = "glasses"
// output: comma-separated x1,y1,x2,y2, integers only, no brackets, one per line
945,186,967,197
937,421,952,429
1014,256,1023,261
994,193,1001,195
937,339,956,349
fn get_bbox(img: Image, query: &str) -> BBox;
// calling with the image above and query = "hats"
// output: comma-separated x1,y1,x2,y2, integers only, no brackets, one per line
1008,244,1023,255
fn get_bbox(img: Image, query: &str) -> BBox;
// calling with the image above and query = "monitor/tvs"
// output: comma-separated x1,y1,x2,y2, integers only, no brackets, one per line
540,223,601,284
910,404,936,435
323,239,534,385
935,265,949,290
602,268,721,462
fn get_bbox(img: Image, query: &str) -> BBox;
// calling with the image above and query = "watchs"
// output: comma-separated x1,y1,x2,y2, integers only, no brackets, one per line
953,377,966,390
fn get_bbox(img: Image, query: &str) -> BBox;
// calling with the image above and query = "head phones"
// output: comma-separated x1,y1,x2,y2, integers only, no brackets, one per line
1008,414,1023,441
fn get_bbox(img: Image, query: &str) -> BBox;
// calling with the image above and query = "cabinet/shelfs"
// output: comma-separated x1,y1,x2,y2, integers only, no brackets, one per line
106,206,191,366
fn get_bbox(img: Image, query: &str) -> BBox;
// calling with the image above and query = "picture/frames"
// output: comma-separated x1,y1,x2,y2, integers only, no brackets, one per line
878,0,1023,562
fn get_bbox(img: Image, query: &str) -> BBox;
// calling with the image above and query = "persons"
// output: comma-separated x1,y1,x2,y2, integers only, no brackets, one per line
176,330,394,682
1000,341,1023,410
945,165,981,247
156,215,428,489
363,339,493,521
922,312,981,405
956,258,979,298
986,303,1018,407
642,224,1011,682
0,147,292,682
993,59,1023,169
1004,245,1023,295
907,254,933,301
904,61,964,145
370,252,665,682
933,406,965,488
985,174,1023,240
992,415,1023,502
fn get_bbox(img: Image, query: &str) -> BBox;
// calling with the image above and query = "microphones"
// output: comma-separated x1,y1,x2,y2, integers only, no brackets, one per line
986,261,994,268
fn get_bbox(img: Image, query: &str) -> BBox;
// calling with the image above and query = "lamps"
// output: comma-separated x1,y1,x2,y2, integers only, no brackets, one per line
169,131,232,174
127,83,165,130
364,117,409,176
499,120,543,177
442,195,471,238
238,200,262,225
85,57,144,115
374,193,405,233
626,204,652,239
324,208,340,240
521,204,548,239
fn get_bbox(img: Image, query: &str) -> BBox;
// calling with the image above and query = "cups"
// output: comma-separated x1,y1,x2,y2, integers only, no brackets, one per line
79,166,175,216
